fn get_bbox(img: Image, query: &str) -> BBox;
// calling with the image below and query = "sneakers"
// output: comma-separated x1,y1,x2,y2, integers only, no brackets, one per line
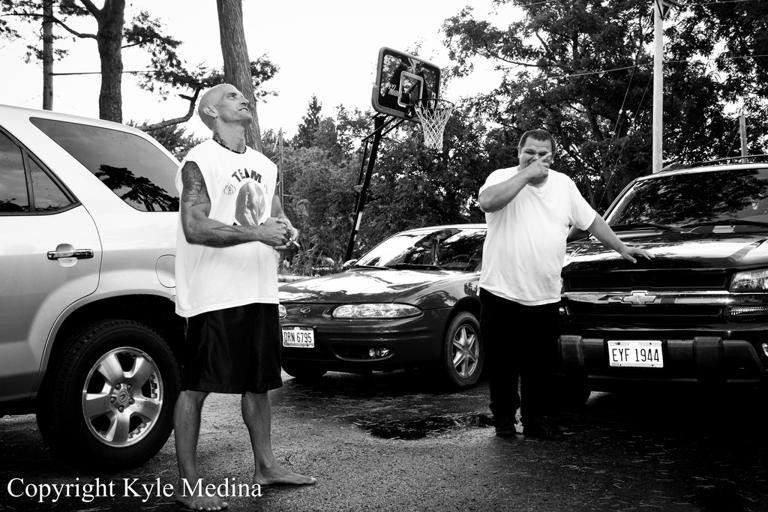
490,415,518,439
520,414,549,443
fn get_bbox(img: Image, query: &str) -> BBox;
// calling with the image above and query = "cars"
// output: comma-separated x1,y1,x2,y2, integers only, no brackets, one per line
278,221,489,387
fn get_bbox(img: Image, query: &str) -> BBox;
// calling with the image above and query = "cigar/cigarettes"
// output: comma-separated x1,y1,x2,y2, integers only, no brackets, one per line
289,238,301,248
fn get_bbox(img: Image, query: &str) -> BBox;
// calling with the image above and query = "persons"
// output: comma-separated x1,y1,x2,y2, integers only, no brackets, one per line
477,129,655,443
175,82,316,511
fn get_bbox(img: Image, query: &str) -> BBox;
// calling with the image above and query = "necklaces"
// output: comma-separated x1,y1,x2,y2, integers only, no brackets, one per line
212,138,246,153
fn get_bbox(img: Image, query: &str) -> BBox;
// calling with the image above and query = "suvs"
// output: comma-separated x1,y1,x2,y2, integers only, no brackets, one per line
1,101,183,470
560,155,767,418
